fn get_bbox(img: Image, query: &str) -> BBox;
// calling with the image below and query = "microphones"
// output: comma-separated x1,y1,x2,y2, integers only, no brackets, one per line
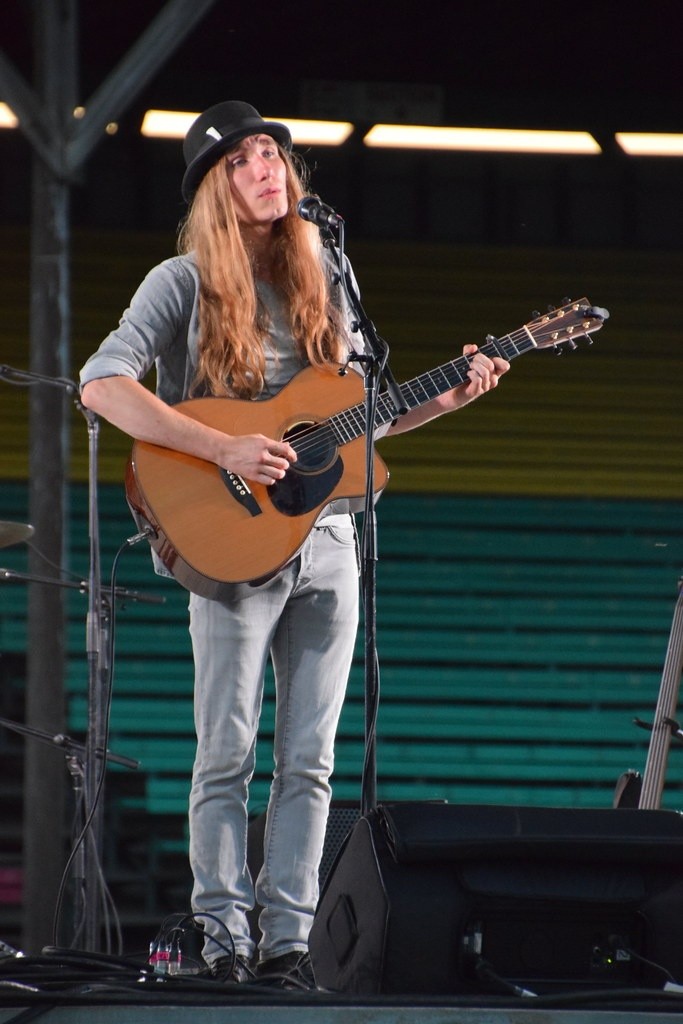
297,196,346,228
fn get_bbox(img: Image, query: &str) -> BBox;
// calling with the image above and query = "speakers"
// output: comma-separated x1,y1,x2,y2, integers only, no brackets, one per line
305,806,682,995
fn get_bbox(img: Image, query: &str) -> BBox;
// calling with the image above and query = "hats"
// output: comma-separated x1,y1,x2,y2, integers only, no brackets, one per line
180,100,292,206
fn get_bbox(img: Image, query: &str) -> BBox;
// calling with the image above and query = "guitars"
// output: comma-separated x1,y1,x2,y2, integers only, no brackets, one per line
123,296,609,601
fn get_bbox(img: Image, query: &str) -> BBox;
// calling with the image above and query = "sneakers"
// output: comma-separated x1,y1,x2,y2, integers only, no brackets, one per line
253,951,317,989
209,954,251,987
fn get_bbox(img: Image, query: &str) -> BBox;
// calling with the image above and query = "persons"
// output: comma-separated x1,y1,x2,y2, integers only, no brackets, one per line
76,98,515,989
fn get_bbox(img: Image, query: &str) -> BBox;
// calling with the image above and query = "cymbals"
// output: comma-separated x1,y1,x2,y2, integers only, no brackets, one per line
0,520,34,549
